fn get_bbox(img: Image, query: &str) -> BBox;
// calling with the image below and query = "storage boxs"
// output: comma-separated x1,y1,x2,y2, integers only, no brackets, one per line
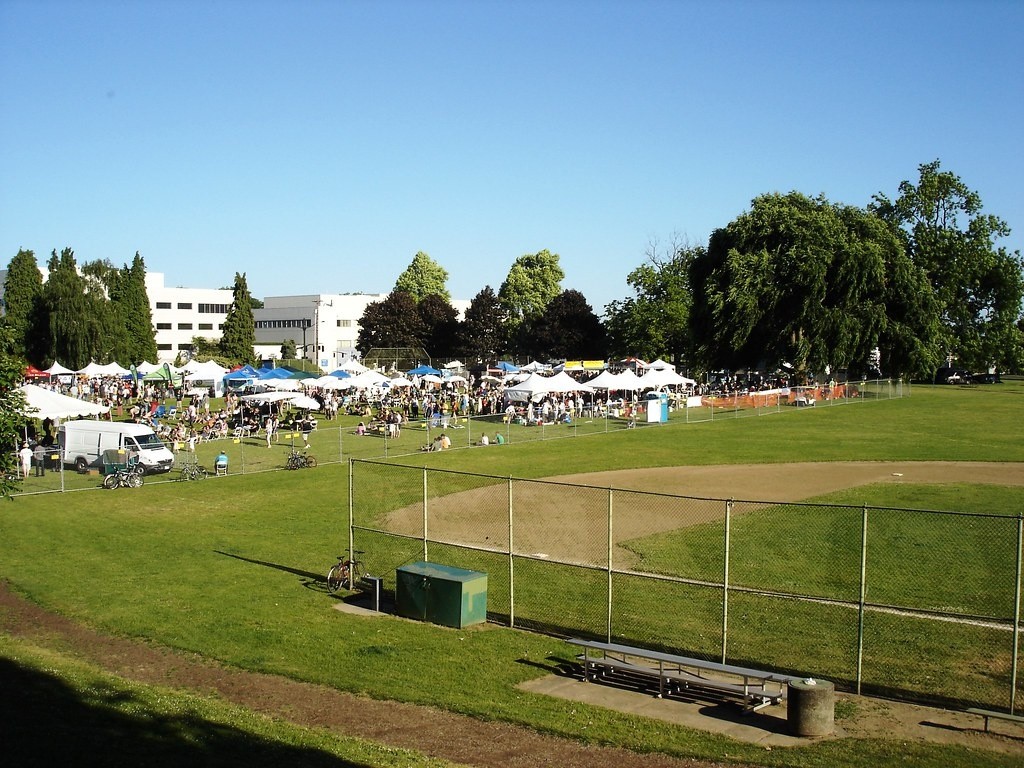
395,562,487,629
87,467,100,474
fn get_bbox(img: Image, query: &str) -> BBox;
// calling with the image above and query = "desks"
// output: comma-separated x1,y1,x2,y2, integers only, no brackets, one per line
283,400,291,410
188,387,211,396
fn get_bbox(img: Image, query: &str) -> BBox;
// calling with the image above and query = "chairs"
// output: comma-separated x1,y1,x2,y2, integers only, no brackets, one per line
154,406,166,418
233,428,241,440
205,429,212,443
167,409,176,420
216,464,228,476
163,406,176,418
253,424,261,435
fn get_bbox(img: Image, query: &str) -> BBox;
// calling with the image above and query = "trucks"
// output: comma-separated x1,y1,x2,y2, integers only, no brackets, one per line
62,419,175,474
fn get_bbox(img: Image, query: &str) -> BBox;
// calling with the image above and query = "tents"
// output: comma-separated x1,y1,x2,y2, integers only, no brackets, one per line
1,359,466,446
479,357,697,403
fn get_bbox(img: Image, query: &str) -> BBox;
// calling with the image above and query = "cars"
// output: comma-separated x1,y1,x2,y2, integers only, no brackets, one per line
947,371,972,385
828,368,848,382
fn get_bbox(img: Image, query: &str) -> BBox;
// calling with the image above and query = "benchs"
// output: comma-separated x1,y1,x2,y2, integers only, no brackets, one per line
565,638,773,713
965,708,1024,733
767,672,801,692
576,656,783,698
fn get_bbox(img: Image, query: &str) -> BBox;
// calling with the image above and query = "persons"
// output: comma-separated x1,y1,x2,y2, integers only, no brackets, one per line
214,451,229,476
16,372,836,455
20,444,34,478
35,442,46,477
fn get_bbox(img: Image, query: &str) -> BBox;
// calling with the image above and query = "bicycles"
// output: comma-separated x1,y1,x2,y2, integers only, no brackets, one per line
285,451,317,470
103,466,144,490
327,549,366,593
179,461,208,482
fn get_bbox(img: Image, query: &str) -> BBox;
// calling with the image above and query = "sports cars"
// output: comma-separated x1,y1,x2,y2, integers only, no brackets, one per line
792,397,816,407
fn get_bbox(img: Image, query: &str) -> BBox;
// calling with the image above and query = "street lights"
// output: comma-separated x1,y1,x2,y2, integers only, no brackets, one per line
303,317,307,358
